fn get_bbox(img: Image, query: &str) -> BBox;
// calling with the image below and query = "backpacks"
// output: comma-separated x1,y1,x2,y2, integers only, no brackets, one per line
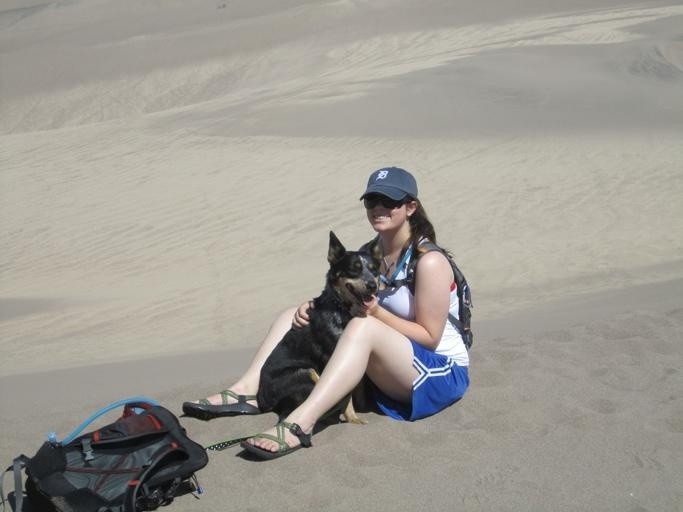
0,397,208,512
371,236,473,350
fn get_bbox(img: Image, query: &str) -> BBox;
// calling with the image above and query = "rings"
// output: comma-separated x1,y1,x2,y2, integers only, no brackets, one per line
295,312,299,315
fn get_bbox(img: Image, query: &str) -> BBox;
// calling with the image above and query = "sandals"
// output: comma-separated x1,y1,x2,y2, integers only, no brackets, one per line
241,419,311,458
183,390,260,415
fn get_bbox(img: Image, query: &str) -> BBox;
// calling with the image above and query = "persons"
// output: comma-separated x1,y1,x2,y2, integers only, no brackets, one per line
182,166,469,462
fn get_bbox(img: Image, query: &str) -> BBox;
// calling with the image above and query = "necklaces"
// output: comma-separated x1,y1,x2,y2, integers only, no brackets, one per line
380,249,397,275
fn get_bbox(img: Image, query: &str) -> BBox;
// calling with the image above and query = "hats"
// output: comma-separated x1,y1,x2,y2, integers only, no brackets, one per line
360,167,417,201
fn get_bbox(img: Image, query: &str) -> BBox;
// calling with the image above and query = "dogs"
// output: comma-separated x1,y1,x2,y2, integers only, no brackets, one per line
256,229,382,424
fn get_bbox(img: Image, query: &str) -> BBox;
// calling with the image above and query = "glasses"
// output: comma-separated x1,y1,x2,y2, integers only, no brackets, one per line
364,197,407,209
